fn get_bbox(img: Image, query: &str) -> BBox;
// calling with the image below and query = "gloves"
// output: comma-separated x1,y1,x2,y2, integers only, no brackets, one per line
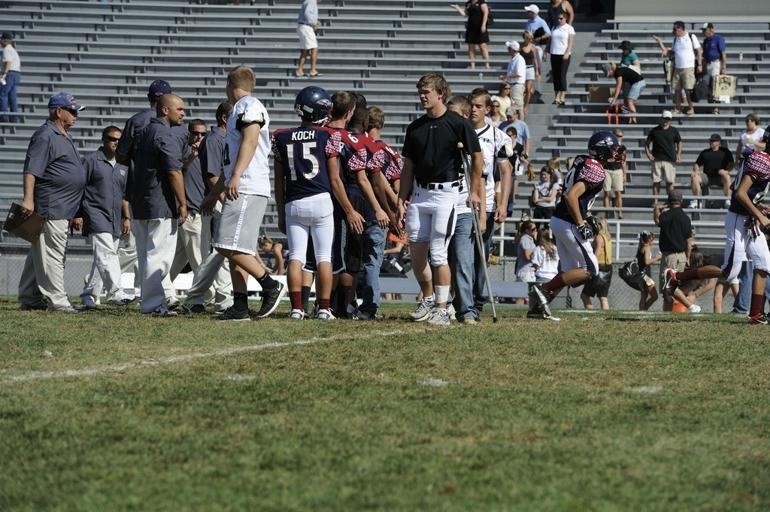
577,221,594,240
587,216,602,236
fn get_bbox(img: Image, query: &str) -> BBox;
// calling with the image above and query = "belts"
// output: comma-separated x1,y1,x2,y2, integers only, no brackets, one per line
417,180,459,190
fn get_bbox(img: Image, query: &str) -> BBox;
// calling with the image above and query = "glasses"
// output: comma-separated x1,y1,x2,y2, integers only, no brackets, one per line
492,105,499,108
106,134,121,142
191,129,207,137
505,87,510,90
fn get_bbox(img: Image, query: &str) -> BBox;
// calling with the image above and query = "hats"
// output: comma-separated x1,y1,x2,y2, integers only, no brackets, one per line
700,22,712,29
506,41,520,51
148,80,171,101
618,41,634,52
662,110,672,119
710,134,721,141
602,63,611,77
505,107,516,117
47,93,86,111
524,4,539,15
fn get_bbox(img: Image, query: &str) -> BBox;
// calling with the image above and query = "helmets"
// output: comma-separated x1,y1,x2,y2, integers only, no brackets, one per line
588,132,619,166
294,87,333,125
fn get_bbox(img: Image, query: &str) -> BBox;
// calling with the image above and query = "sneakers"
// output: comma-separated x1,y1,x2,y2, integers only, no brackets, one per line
211,306,250,321
747,314,769,324
663,268,677,302
532,283,553,316
258,281,286,319
724,199,730,207
20,295,226,317
688,200,703,209
688,305,700,313
289,304,378,321
527,307,560,322
410,297,480,325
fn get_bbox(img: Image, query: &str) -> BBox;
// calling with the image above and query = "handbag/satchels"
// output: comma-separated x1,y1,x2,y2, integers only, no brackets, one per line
618,258,646,292
692,80,709,102
665,59,675,84
712,75,735,103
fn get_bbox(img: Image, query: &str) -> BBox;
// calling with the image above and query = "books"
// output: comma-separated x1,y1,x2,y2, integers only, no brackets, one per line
4,200,43,245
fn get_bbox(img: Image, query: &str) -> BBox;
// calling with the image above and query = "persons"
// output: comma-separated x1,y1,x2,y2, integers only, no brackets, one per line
19,1,767,328
0,31,20,122
